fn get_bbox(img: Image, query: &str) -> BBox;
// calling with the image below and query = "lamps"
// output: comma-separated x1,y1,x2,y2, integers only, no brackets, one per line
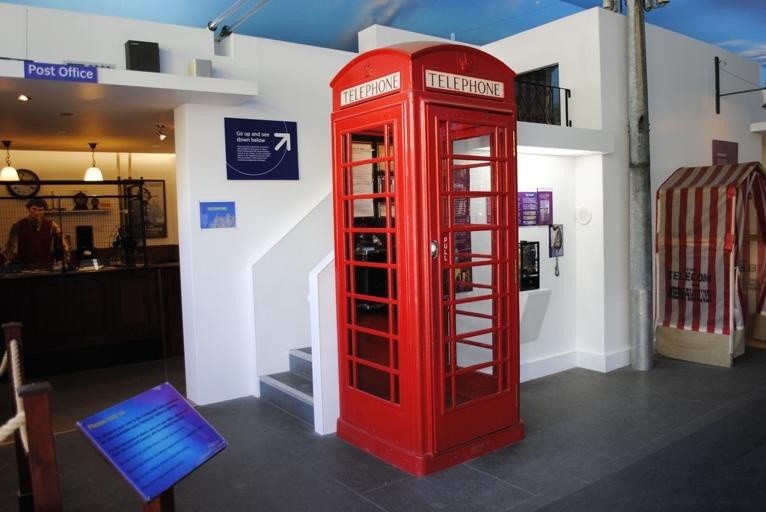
84,143,103,181
0,140,19,182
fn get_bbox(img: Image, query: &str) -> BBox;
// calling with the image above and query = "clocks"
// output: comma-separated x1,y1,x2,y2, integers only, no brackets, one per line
6,169,40,200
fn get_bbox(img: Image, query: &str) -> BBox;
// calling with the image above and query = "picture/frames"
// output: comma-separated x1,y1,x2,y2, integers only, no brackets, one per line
123,179,167,239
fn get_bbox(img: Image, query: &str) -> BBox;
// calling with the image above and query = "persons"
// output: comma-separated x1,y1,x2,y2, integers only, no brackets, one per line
0,196,72,272
